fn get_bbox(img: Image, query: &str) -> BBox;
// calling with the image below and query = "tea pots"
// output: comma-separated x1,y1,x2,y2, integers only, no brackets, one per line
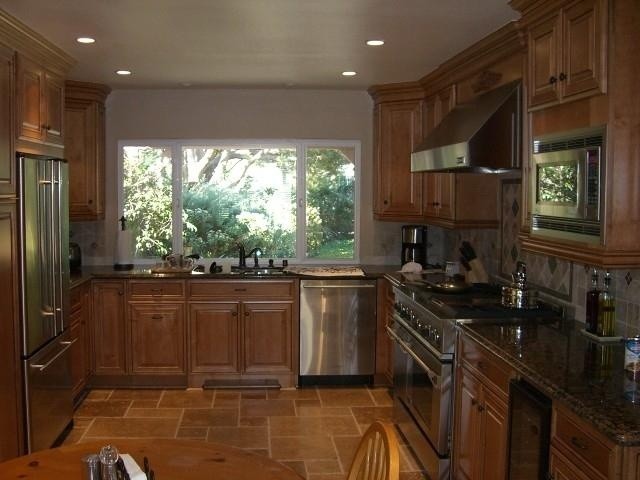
502,261,541,310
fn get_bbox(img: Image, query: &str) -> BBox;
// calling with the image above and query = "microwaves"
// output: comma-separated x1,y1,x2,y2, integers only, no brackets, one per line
529,147,601,220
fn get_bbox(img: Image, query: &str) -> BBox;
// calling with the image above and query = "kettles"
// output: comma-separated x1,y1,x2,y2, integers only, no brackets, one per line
68,242,81,270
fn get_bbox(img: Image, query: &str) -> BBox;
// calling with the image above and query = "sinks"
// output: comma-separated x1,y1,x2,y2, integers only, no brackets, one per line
192,268,289,276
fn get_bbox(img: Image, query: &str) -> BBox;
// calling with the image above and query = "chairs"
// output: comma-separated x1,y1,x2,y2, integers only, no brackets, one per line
345,421,402,480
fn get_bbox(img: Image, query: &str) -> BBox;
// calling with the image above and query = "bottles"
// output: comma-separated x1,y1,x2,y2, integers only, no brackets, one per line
586,270,616,336
100,444,120,480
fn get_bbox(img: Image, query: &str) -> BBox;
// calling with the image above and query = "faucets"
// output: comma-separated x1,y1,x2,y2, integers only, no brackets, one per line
237,243,263,269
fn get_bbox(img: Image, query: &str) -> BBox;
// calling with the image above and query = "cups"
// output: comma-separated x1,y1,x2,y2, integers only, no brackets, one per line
81,453,101,480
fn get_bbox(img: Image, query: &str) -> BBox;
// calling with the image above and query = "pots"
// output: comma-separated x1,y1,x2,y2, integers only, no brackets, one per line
416,278,473,295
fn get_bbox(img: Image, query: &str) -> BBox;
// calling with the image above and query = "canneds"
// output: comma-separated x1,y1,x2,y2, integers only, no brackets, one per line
623,337,640,374
623,370,639,406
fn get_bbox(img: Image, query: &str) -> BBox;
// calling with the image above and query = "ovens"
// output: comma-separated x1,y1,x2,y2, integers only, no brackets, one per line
385,317,454,457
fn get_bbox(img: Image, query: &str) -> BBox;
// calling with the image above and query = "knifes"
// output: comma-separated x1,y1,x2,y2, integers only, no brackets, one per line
459,239,475,271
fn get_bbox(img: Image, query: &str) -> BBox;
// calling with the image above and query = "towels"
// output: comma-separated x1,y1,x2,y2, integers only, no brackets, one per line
401,262,422,274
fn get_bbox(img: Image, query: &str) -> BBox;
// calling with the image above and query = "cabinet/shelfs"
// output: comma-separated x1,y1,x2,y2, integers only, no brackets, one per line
526,0,608,111
422,83,498,227
189,279,294,375
125,279,186,378
453,333,517,480
0,8,24,461
91,280,125,375
15,51,65,149
550,398,623,480
65,81,112,221
381,99,421,217
70,276,90,397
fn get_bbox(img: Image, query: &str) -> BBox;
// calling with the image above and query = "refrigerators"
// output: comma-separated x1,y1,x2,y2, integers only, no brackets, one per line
17,151,76,455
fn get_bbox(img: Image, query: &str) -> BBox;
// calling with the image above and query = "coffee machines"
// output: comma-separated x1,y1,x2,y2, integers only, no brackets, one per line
400,225,432,268
